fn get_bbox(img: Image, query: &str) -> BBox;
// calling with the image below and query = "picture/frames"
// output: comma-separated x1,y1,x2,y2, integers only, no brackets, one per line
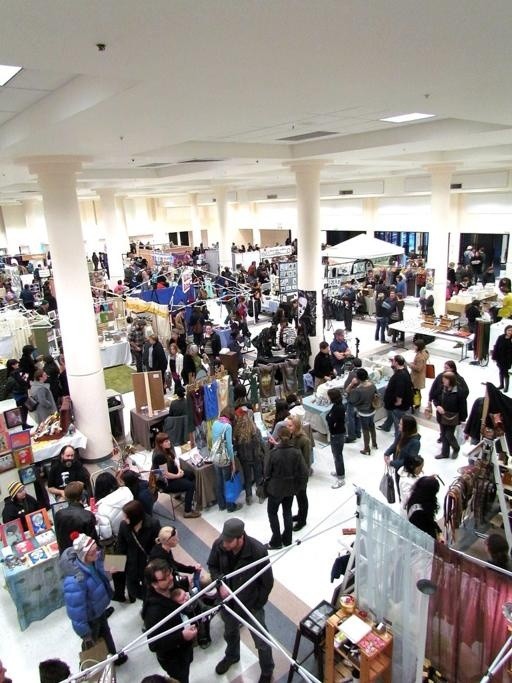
0,407,98,586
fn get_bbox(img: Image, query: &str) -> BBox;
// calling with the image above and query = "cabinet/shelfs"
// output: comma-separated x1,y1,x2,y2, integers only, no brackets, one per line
323,609,394,683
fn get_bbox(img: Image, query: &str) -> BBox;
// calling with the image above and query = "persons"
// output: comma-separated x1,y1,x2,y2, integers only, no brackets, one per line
2,239,512,683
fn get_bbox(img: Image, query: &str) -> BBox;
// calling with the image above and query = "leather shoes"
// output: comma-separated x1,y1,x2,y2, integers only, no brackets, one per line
184,511,201,518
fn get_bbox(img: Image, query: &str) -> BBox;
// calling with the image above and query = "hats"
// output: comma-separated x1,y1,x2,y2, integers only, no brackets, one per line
7,480,24,498
220,518,244,541
70,532,95,563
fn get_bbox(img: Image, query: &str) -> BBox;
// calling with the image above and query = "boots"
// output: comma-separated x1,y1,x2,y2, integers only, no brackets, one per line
370,428,378,449
452,445,460,459
360,429,370,455
436,448,450,459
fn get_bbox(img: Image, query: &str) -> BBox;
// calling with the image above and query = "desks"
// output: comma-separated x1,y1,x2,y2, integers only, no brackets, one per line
99,336,130,369
302,372,392,432
178,445,253,513
388,317,474,363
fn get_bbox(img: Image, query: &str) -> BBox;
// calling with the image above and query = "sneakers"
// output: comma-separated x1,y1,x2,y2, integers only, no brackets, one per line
258,671,271,682
376,425,383,429
246,471,345,549
217,659,232,673
344,436,356,442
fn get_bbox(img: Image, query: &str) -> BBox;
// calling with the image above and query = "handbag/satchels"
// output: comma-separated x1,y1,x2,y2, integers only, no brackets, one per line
99,535,122,561
80,637,109,670
413,387,421,407
209,438,231,467
24,397,38,412
426,365,435,378
225,470,243,502
441,411,459,425
379,473,396,503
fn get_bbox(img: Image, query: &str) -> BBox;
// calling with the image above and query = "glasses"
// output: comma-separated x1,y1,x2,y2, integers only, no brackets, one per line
168,526,176,541
